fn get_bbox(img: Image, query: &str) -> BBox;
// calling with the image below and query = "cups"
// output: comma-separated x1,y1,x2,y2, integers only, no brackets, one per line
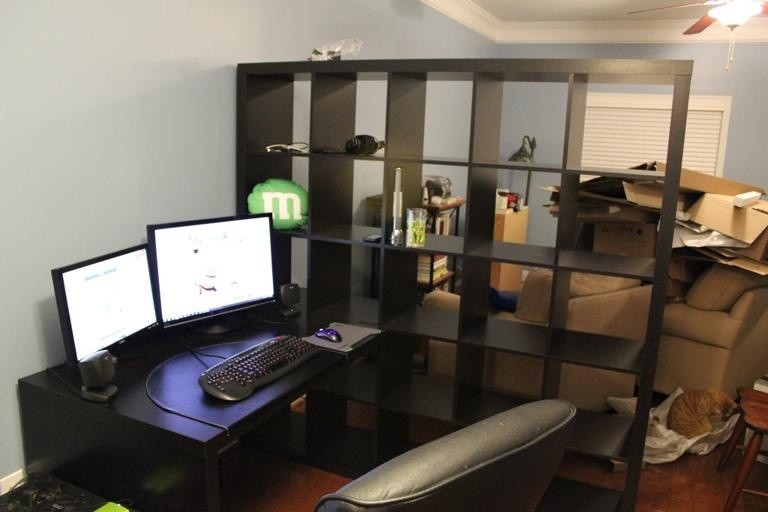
406,206,426,248
496,188,508,210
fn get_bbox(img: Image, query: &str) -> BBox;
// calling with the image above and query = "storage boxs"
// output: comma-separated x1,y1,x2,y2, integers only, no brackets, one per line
535,161,768,277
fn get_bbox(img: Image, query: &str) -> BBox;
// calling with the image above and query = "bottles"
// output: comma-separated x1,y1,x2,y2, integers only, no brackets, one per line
345,135,386,155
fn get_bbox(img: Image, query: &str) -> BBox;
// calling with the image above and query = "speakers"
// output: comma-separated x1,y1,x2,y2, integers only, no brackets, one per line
79,350,119,403
278,282,302,318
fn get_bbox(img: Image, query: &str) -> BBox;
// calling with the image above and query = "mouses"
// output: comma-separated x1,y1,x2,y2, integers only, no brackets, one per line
315,327,342,343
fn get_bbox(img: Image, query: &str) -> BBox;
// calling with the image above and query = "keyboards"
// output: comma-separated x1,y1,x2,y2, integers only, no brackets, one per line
198,333,327,403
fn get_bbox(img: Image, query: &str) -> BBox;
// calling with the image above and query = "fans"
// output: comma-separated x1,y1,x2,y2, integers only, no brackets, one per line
627,1,768,36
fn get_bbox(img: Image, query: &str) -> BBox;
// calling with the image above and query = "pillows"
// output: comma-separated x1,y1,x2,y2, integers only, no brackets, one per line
515,267,642,323
687,263,767,311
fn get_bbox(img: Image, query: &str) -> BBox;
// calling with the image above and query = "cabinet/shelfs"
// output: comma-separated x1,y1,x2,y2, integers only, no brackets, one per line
367,193,467,374
237,58,694,512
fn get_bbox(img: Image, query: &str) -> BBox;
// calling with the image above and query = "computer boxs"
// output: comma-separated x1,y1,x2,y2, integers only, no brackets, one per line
1,470,141,512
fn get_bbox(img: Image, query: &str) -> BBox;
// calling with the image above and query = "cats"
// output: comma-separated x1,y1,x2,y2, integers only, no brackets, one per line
667,388,742,439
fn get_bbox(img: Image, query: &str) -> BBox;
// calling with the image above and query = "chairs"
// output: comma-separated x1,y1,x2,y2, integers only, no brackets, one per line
421,284,654,413
718,385,766,510
314,397,578,511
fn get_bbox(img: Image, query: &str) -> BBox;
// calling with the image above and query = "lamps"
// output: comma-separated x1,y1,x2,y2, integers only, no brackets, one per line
707,3,764,71
509,136,537,206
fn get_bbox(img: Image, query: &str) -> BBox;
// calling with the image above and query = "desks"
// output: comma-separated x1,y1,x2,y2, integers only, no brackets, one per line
490,205,529,292
17,302,393,512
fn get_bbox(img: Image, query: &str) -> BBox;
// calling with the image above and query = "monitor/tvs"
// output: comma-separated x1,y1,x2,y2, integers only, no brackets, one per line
146,210,281,336
50,243,162,365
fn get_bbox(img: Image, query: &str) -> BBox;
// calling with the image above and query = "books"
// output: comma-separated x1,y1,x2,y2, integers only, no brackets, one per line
412,254,450,282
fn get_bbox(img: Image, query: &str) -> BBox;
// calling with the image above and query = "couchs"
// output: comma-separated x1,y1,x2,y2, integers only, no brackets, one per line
635,265,767,402
